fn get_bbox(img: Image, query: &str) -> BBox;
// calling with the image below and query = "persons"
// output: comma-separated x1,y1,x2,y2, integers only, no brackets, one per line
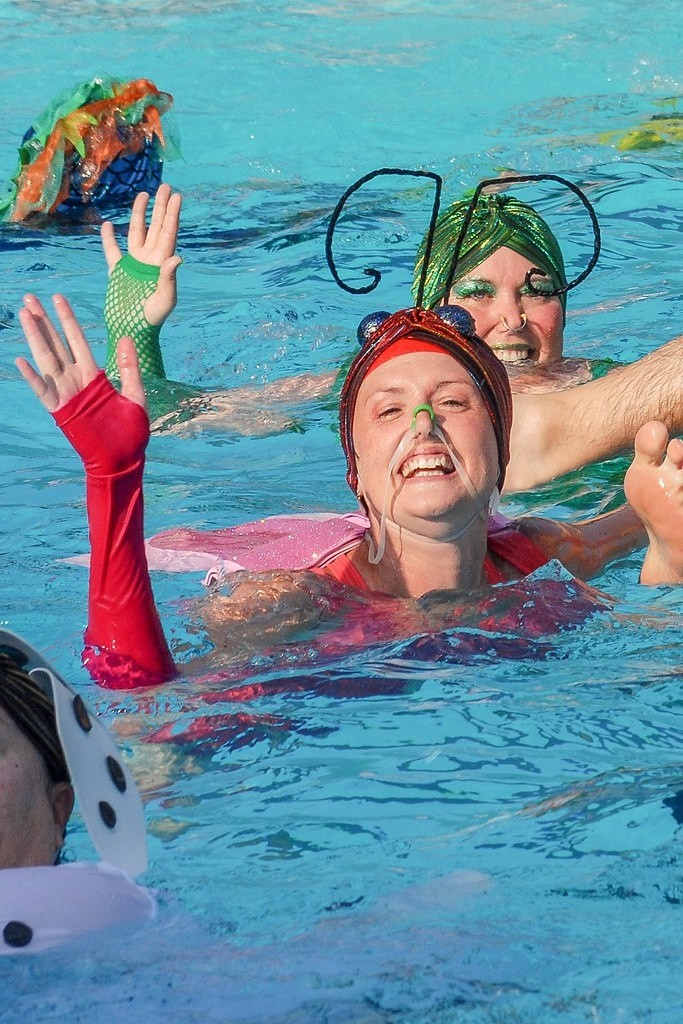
96,184,637,439
499,330,682,590
0,632,162,959
15,289,614,697
